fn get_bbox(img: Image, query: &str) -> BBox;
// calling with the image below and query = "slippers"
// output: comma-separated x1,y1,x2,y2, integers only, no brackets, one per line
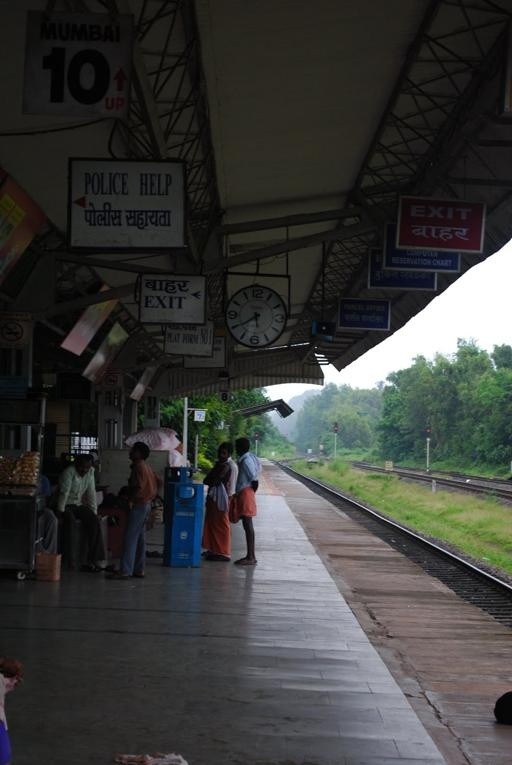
234,556,257,565
202,550,231,561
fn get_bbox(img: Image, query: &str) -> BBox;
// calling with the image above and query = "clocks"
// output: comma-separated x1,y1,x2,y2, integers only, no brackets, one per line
225,284,288,348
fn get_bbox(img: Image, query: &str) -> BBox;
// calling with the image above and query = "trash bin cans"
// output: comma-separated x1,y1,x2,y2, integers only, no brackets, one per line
164,467,204,567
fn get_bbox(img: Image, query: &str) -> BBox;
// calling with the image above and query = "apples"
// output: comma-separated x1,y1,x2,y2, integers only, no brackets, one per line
0,452,40,485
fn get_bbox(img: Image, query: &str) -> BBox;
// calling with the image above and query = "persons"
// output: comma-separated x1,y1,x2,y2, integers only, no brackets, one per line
202,442,239,561
49,455,107,570
40,476,58,554
105,441,158,580
231,437,260,566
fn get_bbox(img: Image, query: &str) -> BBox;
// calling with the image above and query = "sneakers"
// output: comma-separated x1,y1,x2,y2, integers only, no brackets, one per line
106,571,144,579
94,559,107,569
80,563,100,573
